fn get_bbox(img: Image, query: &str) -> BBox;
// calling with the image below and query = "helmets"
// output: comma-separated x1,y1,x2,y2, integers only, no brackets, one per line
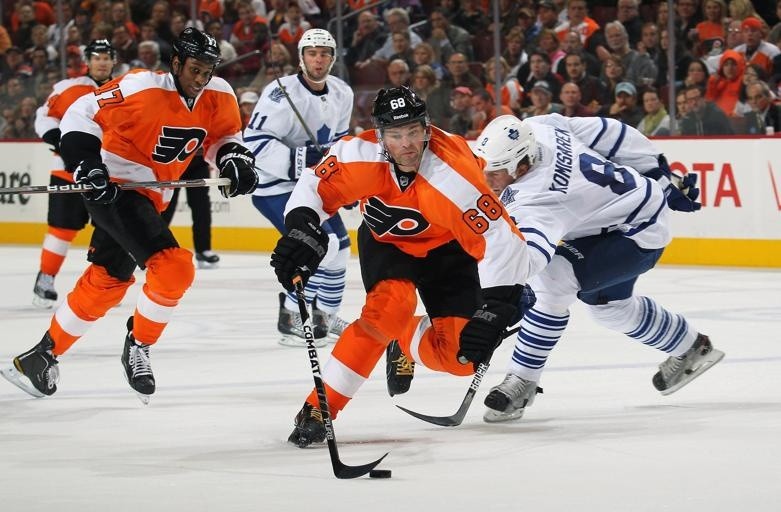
85,38,114,53
172,27,220,67
298,28,338,58
474,115,535,173
372,86,427,129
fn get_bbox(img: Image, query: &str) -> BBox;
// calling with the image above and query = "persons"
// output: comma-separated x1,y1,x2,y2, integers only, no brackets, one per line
243,28,359,348
31,37,119,310
472,113,725,422
1,27,259,404
159,146,220,269
2,1,780,142
269,83,531,448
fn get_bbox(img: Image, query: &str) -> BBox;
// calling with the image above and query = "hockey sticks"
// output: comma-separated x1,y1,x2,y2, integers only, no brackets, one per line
396,327,520,426
213,49,264,72
289,269,390,479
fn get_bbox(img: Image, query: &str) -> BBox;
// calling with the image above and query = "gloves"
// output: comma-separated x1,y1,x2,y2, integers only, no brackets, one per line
647,158,701,212
271,206,329,293
59,130,122,204
457,284,523,370
215,142,259,196
517,282,540,327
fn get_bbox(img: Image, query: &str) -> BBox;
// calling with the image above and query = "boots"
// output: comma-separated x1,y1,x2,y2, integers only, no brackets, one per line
32,271,57,300
652,332,713,390
277,291,328,338
387,340,413,394
485,373,538,413
13,328,61,397
312,293,353,339
122,314,156,395
194,247,223,263
294,399,327,441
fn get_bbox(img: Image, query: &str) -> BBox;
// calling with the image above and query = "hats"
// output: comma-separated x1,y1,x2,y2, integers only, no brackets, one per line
531,79,555,96
616,82,636,96
